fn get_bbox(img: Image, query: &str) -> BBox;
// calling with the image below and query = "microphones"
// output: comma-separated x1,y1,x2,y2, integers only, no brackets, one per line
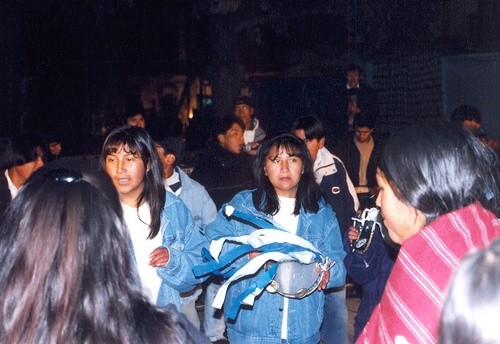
243,144,258,150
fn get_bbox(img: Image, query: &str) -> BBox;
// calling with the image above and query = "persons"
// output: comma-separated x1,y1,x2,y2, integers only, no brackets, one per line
353,116,500,344
0,155,214,344
205,130,347,344
0,58,499,298
189,114,259,344
99,125,212,333
289,114,360,344
